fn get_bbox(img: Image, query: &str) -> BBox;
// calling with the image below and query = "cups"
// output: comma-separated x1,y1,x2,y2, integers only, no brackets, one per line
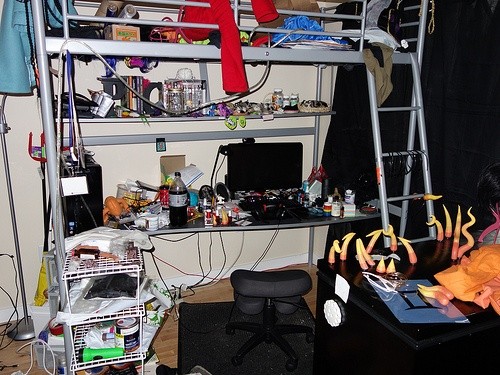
159,184,170,210
117,183,143,201
297,176,356,218
145,214,159,230
188,191,198,206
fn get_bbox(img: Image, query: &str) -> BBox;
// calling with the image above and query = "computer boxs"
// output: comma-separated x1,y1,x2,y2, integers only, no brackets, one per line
59,153,104,238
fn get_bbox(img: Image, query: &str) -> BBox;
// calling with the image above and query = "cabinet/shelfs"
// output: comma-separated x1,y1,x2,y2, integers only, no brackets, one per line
61,245,147,375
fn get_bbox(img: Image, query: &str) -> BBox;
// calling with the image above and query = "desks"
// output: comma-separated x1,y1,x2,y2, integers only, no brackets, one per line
138,205,380,237
316,237,500,375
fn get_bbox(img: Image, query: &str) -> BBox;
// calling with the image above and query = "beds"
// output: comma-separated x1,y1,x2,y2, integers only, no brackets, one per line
31,0,438,375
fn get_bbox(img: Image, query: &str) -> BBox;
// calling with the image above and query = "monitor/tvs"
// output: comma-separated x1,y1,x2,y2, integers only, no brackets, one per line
226,143,303,190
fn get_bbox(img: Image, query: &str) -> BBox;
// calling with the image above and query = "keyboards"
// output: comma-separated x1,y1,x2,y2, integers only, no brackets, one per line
239,199,300,210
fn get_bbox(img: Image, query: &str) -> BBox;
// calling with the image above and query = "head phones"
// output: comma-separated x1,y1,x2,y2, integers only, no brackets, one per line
252,203,293,220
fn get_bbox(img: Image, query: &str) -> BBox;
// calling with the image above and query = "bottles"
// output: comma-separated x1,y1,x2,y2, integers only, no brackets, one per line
290,96,298,106
292,92,300,103
272,89,283,108
283,96,290,108
166,88,184,117
167,172,188,227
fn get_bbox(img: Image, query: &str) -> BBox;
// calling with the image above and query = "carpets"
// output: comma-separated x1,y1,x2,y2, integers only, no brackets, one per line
177,299,316,375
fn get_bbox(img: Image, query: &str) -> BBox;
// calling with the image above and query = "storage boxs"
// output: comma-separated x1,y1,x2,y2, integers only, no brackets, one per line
105,25,141,42
30,301,66,369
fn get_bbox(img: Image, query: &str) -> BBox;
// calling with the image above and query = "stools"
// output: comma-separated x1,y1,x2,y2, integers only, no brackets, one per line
226,269,314,372
